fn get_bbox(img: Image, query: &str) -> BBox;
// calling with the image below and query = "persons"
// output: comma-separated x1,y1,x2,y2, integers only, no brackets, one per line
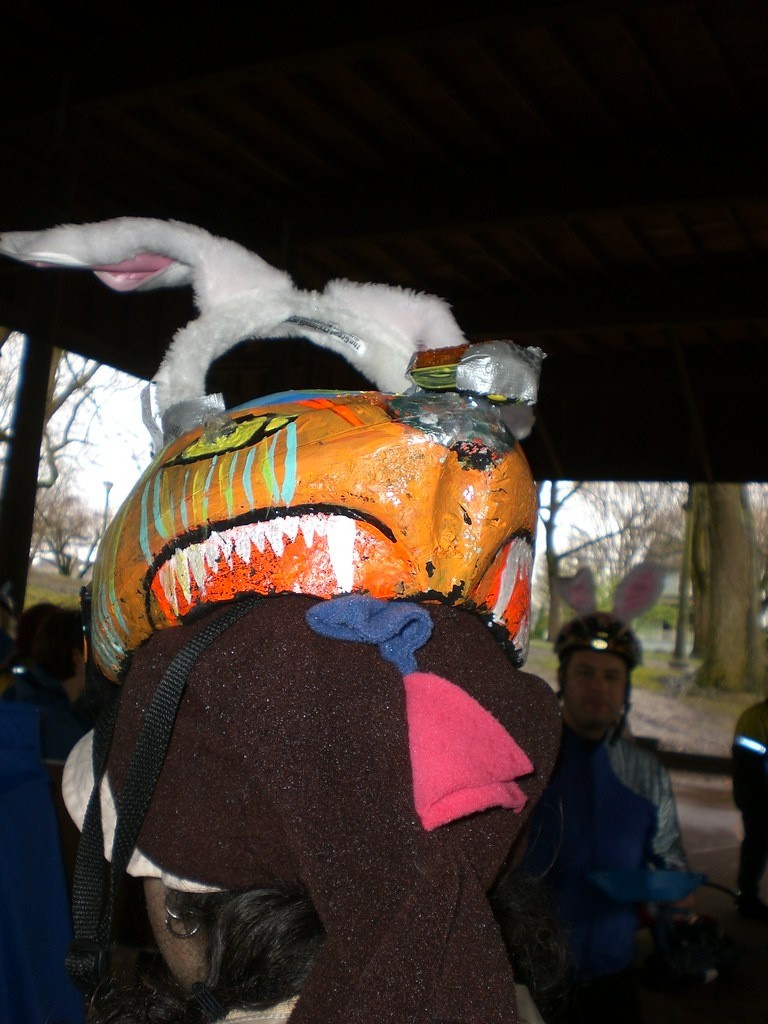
2,600,768,1023
1,216,564,1024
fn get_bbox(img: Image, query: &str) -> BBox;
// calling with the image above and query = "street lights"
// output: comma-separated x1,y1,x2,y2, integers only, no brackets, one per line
102,481,112,538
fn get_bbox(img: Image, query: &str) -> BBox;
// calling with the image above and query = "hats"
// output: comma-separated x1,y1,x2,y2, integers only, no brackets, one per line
62,587,566,1024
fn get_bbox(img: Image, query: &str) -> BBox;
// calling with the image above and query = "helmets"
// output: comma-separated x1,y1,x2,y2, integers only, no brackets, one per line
548,616,647,666
